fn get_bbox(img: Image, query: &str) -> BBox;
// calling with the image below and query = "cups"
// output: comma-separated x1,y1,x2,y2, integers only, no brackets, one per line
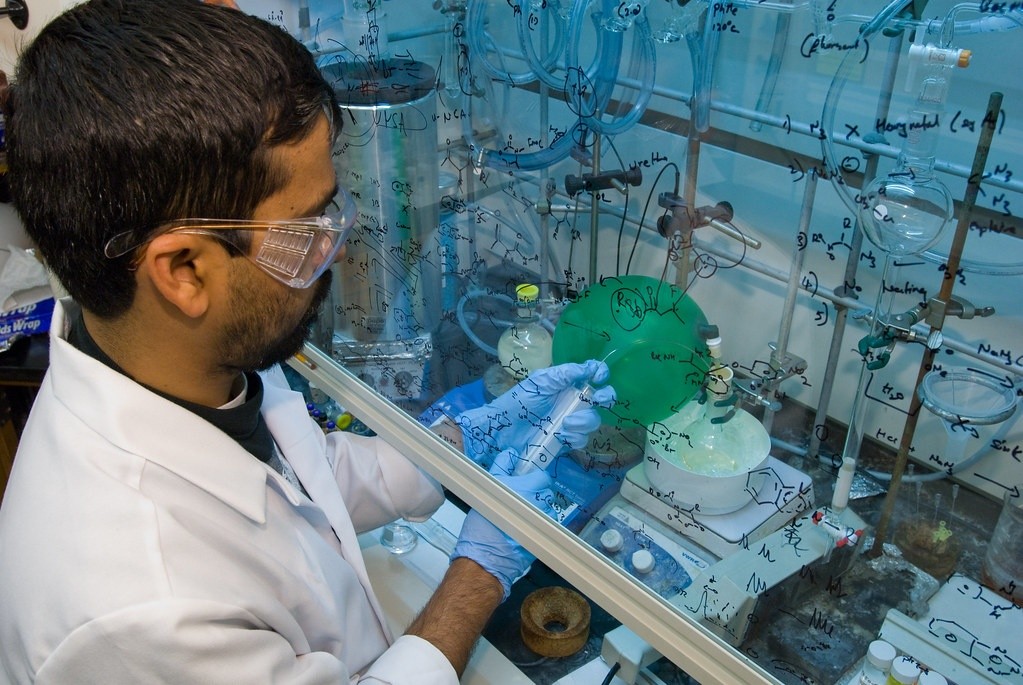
984,486,1023,600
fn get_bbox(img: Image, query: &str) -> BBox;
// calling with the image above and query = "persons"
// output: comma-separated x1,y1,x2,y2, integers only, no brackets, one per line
0,0,615,685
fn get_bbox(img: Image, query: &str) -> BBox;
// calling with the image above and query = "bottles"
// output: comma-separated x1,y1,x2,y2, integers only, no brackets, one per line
858,640,948,685
498,284,552,381
680,364,747,477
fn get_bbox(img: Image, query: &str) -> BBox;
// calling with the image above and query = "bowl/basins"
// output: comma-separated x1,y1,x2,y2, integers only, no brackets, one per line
643,400,771,516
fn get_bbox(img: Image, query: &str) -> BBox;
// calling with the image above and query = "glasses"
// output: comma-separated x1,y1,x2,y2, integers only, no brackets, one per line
103,180,357,288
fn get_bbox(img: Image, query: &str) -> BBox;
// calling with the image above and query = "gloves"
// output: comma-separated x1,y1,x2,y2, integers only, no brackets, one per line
456,357,615,473
450,447,559,606
